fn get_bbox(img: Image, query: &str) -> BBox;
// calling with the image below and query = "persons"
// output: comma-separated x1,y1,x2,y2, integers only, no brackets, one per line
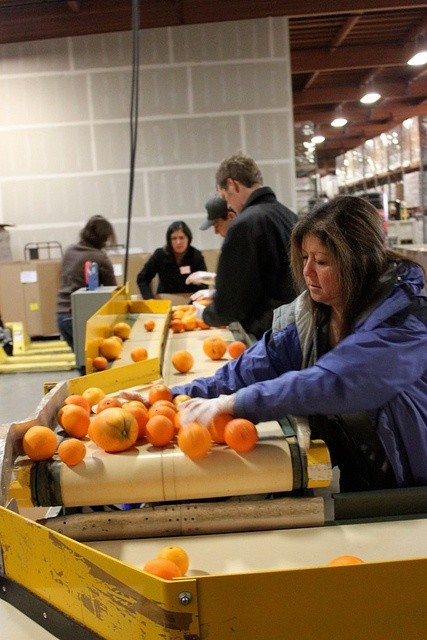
91,193,427,494
193,151,307,341
184,193,239,301
55,214,118,354
135,221,209,301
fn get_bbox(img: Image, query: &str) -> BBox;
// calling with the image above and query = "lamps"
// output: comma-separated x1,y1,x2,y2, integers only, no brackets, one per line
330,105,349,130
405,37,427,67
358,72,381,105
309,124,327,147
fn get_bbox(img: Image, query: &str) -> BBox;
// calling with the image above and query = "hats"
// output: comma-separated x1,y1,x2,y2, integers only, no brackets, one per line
199,196,227,231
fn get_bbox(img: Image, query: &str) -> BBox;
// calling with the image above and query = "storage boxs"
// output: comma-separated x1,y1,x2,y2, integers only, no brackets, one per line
107,253,147,297
0,260,63,338
200,248,221,277
